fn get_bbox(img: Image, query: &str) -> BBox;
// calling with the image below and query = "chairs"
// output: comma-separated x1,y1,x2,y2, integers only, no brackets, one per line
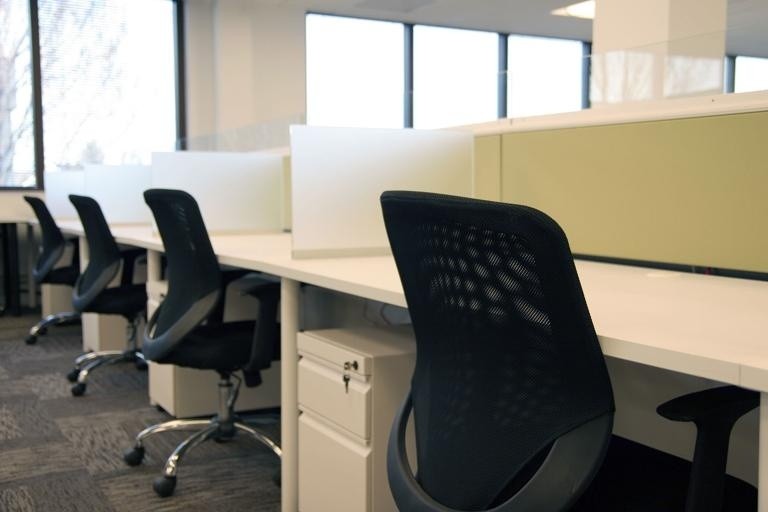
67,193,147,399
379,189,760,511
120,189,307,498
23,194,81,346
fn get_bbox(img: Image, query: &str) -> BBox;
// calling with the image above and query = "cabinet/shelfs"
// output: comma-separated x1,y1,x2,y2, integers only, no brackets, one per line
148,273,282,418
31,241,146,360
293,320,418,512
